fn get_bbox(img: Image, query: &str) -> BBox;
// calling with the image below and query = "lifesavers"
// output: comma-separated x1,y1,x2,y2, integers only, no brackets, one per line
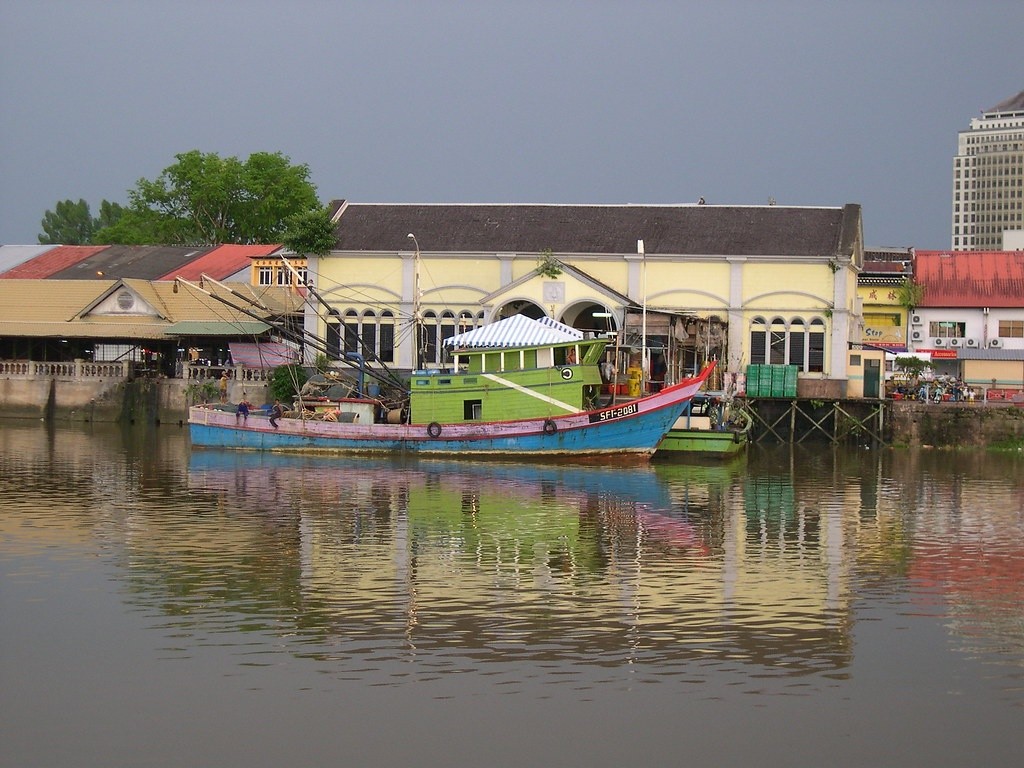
426,422,442,438
543,420,558,436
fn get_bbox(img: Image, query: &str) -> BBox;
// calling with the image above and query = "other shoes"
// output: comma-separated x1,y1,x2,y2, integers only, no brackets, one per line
221,404,228,407
274,425,278,430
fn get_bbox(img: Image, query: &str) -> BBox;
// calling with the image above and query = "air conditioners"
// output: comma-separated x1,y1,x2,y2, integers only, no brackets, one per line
935,338,947,346
912,330,923,341
990,338,1003,347
911,314,923,325
965,338,978,347
950,339,963,347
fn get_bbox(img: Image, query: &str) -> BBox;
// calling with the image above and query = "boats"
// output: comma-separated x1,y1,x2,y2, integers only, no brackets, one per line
172,254,719,464
662,385,756,461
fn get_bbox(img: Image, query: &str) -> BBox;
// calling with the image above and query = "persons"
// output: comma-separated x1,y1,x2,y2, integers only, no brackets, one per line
896,372,976,405
220,371,229,404
269,399,284,432
566,348,582,365
236,399,249,426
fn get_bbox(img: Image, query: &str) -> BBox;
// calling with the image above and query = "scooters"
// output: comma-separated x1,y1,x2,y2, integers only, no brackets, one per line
918,379,944,404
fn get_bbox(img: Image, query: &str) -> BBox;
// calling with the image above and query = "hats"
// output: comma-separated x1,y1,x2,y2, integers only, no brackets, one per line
222,371,228,374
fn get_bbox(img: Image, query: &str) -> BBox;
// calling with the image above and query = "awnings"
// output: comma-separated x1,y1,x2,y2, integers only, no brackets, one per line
957,348,1024,361
164,322,273,336
848,340,897,355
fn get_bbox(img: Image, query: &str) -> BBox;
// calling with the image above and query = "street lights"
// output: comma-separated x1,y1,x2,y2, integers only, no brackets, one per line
636,238,647,398
408,231,416,375
